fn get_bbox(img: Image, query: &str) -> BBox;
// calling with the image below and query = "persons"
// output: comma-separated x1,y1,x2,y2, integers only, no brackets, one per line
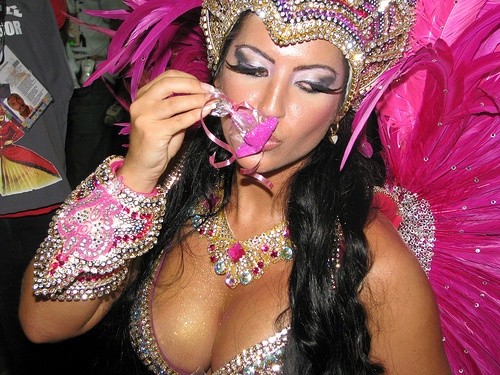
20,1,453,375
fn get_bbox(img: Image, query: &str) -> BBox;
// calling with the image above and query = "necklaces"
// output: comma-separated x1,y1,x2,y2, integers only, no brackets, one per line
191,186,296,286
